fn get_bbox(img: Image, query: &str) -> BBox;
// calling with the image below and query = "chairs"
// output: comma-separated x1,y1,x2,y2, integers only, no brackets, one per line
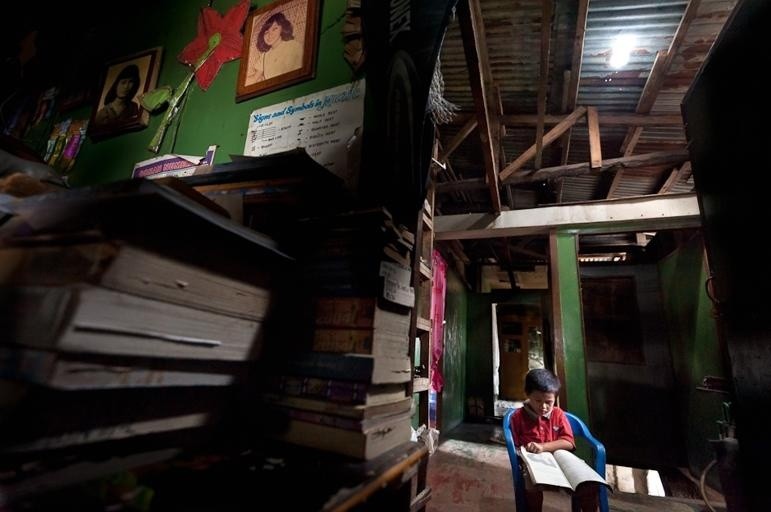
502,406,612,511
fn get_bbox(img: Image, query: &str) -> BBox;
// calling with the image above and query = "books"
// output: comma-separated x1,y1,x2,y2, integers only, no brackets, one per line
2,147,414,509
518,442,614,494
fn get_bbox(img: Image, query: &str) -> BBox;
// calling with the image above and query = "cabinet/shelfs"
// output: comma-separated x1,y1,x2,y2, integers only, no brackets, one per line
399,122,447,511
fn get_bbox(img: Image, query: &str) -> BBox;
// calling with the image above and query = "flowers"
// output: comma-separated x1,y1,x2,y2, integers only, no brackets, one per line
137,1,251,154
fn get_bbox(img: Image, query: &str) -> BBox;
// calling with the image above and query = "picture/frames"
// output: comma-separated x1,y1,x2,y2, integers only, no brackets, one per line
86,43,162,144
234,0,324,105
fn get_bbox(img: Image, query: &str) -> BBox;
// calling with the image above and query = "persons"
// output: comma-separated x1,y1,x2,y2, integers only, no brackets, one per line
510,365,603,510
249,8,307,80
95,64,141,124
